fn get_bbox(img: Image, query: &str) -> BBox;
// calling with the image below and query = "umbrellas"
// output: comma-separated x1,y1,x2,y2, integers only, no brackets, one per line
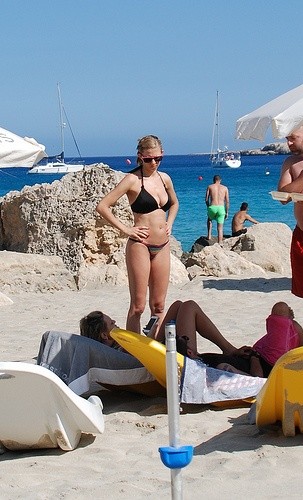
233,85,303,141
0,127,48,168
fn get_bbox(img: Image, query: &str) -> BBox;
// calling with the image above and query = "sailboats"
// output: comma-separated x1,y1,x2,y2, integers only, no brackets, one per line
208,88,243,168
25,82,90,175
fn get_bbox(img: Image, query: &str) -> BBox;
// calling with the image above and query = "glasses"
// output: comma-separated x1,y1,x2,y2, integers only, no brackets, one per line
140,156,164,163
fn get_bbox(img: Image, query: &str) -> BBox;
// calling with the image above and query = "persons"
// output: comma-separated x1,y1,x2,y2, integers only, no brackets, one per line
278,126,303,297
96,134,179,339
205,175,229,243
232,202,260,237
162,301,303,377
80,300,238,356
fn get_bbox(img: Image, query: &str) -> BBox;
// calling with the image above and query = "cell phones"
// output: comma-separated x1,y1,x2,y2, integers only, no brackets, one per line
144,316,159,332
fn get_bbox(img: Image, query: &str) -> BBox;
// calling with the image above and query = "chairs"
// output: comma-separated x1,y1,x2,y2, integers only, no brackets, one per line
37,330,157,399
0,361,104,455
255,344,303,438
109,328,268,410
